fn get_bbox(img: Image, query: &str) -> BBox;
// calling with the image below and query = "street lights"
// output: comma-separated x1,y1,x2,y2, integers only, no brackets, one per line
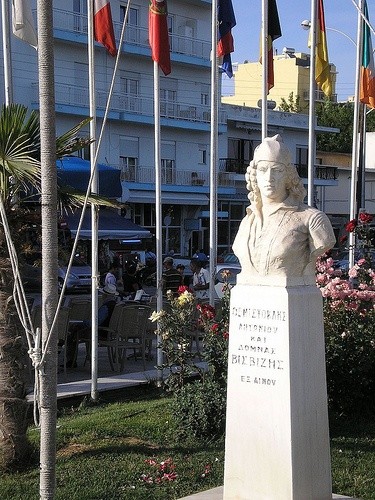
302,17,368,212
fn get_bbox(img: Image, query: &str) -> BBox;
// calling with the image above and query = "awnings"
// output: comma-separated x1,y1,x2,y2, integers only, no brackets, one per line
11,151,124,201
59,208,153,240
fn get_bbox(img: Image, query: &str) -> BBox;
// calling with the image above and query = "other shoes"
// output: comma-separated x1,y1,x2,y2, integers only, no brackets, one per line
60,360,78,368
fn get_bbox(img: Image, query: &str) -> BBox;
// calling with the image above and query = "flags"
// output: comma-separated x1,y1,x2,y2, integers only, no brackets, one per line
215,0,238,80
9,0,39,52
256,0,283,97
358,1,375,111
145,0,172,77
306,0,335,98
93,0,118,59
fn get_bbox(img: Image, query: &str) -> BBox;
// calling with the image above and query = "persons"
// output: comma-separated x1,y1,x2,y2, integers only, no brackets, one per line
144,248,156,260
59,284,118,369
231,139,336,279
197,248,207,261
123,262,133,274
123,266,142,292
191,248,200,258
99,261,127,302
176,264,186,286
189,259,210,298
162,256,182,298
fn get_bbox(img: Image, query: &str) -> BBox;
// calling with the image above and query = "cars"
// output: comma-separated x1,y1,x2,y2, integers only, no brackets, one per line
149,249,244,298
324,247,375,278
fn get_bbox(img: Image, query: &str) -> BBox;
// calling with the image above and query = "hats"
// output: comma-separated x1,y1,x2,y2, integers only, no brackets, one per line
163,257,174,263
99,284,121,296
253,134,293,165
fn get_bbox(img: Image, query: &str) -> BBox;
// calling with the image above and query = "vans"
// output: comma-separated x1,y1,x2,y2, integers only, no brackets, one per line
20,250,93,292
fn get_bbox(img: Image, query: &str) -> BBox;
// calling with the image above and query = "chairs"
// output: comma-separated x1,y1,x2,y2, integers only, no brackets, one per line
12,290,206,383
